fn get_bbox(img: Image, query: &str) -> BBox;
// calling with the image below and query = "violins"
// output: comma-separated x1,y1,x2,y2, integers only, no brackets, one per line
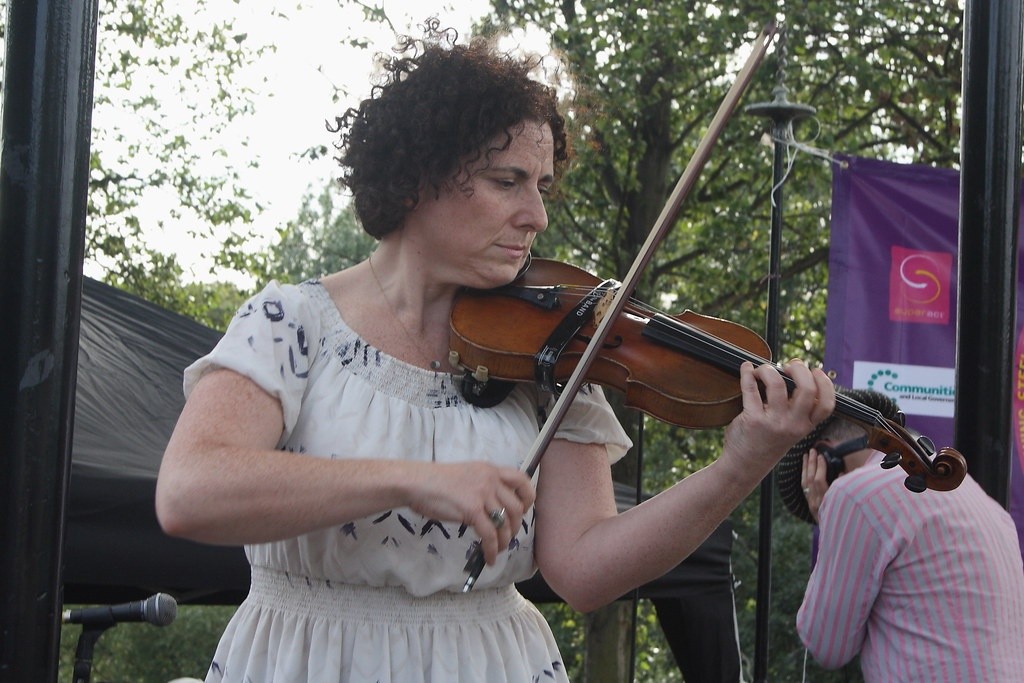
449,256,968,493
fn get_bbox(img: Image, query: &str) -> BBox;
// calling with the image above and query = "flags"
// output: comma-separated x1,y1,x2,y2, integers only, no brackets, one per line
810,151,964,573
1009,179,1024,562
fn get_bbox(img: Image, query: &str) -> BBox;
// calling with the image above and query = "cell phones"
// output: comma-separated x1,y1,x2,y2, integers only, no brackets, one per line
821,448,843,485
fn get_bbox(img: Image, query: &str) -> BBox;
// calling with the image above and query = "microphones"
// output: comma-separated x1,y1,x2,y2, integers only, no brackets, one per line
64,591,178,628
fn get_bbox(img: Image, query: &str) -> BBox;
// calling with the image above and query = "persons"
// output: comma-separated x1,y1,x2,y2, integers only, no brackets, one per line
155,18,836,683
778,389,1024,683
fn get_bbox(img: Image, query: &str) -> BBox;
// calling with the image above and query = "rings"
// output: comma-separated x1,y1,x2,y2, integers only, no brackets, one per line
488,507,506,529
802,487,809,493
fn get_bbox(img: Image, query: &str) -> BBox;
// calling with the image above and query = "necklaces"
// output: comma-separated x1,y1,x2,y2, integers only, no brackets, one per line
369,256,449,370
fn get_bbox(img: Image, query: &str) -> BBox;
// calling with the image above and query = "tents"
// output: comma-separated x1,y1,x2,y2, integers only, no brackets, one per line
62,273,744,683
